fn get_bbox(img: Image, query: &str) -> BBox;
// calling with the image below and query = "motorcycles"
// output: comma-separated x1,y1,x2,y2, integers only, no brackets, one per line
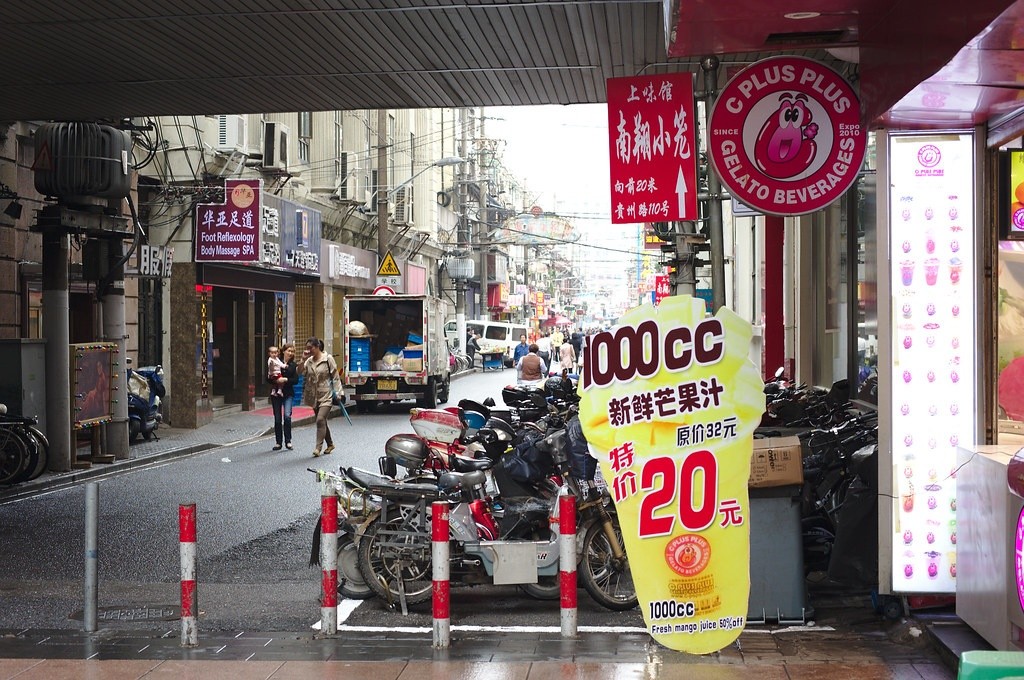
2,277,882,643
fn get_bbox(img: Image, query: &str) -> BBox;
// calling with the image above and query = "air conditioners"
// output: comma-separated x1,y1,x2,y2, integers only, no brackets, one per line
339,151,359,204
393,185,415,227
215,114,250,157
261,121,294,178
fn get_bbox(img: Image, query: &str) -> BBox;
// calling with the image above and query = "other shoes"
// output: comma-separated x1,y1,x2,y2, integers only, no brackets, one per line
285,442,293,449
273,443,282,450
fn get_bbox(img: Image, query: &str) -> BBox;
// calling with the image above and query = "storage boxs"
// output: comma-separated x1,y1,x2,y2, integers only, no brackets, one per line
349,338,371,372
386,330,424,373
747,436,804,489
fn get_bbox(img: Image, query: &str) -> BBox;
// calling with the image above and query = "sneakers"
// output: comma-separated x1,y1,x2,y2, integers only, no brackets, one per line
324,446,334,454
313,451,320,457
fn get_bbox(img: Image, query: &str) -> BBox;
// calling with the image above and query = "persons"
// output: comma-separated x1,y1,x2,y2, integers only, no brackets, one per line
79,357,109,419
512,326,602,382
299,338,345,457
270,343,303,451
467,327,482,367
267,346,287,398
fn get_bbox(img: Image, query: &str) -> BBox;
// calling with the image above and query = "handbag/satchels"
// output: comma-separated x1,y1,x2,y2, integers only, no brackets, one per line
332,395,346,405
